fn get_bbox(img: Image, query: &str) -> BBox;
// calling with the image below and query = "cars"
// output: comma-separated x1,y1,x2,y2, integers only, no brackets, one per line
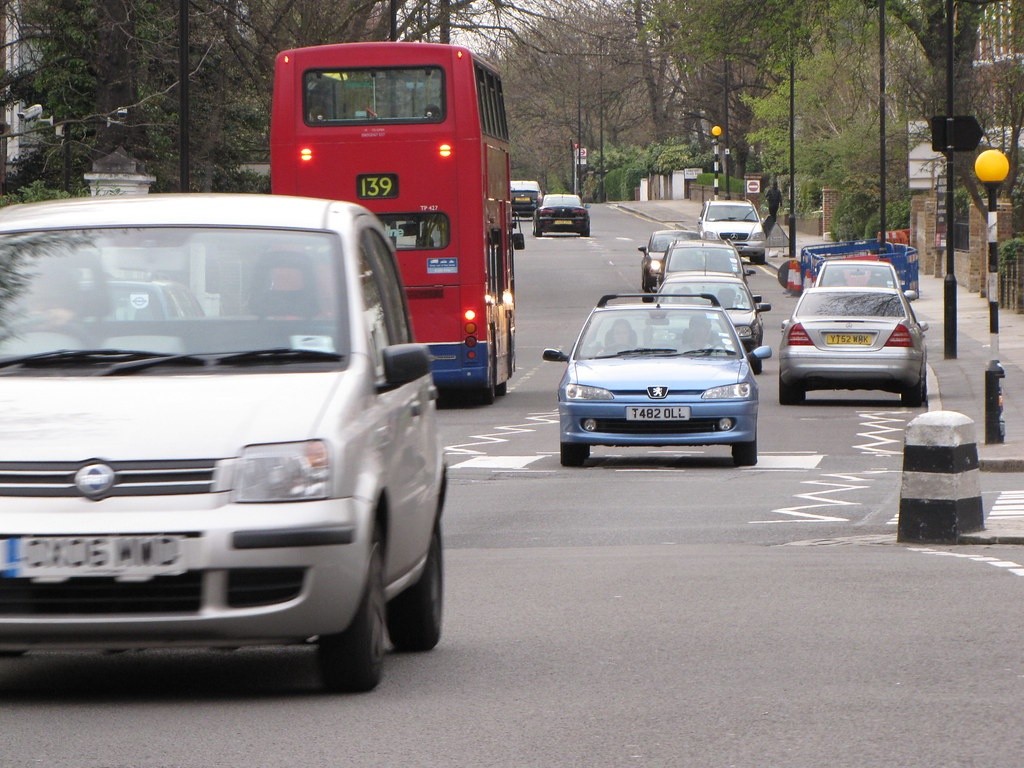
532,194,592,237
643,274,771,377
780,285,931,408
638,230,707,300
510,180,545,218
814,260,919,308
0,192,450,694
541,293,773,465
79,278,206,321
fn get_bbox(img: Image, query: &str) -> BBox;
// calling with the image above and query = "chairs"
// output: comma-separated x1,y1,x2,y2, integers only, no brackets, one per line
682,328,719,352
670,287,693,303
604,328,637,356
866,270,887,287
820,269,846,287
718,287,736,308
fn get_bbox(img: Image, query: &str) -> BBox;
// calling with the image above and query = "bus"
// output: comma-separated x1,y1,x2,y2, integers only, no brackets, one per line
270,43,527,405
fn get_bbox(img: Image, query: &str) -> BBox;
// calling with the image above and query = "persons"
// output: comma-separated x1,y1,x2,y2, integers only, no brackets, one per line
680,315,722,352
766,181,782,223
595,318,637,356
425,104,439,117
515,236,523,248
680,287,692,304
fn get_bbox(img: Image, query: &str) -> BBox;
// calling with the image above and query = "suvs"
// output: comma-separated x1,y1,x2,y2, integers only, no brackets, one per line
703,199,768,265
657,239,756,294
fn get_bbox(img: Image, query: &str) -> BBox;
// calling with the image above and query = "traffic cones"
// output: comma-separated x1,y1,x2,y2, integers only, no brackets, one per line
802,268,818,292
791,268,805,298
782,261,799,296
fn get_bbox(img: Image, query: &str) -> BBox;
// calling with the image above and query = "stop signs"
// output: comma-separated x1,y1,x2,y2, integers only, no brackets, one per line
746,179,760,194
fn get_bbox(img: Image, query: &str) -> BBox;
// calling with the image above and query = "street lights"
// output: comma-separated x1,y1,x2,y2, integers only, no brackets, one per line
710,125,722,201
975,150,1011,445
